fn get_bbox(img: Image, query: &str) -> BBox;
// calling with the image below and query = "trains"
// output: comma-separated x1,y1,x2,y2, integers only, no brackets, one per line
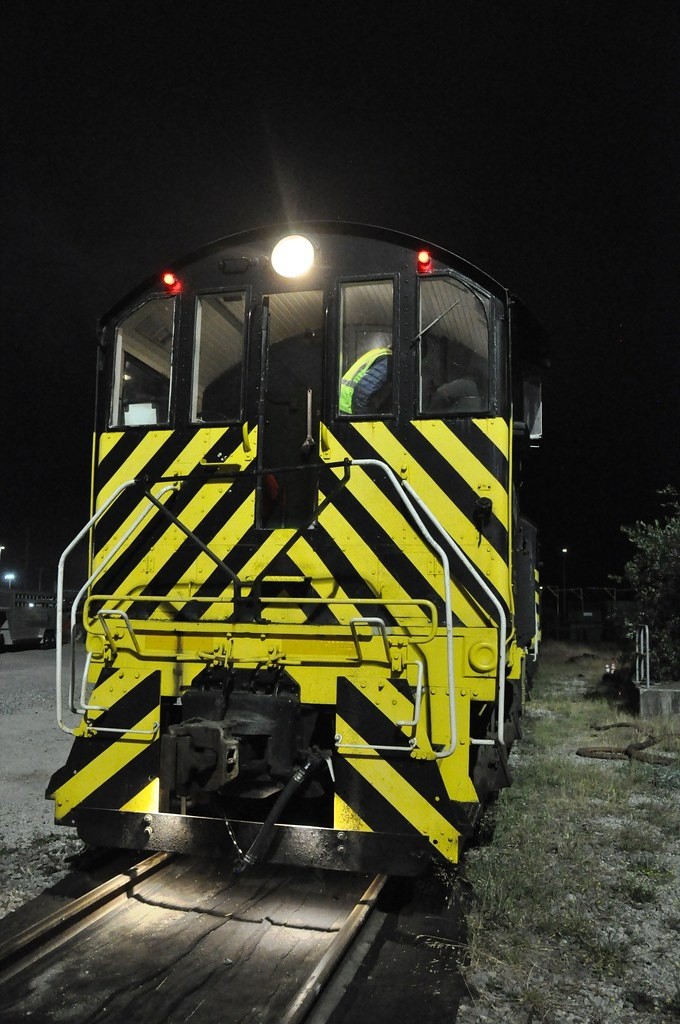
45,211,545,874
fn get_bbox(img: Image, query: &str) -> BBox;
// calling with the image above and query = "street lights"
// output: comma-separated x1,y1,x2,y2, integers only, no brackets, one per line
36,567,45,593
50,575,56,592
3,573,16,589
562,549,568,614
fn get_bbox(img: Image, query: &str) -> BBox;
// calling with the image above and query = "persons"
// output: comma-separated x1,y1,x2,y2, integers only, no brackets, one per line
339,332,428,416
